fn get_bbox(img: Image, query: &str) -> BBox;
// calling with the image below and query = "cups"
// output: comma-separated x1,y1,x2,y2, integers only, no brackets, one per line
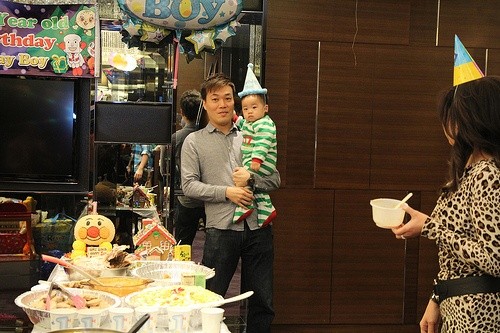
167,306,193,332
77,309,102,328
201,307,225,333
139,186,154,206
109,307,134,330
134,306,158,333
50,309,75,331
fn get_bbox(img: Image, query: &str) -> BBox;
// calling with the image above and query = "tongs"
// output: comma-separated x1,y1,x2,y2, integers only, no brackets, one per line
46,281,86,310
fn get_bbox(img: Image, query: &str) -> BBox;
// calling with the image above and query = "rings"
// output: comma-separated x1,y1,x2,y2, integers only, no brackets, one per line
401,234,406,239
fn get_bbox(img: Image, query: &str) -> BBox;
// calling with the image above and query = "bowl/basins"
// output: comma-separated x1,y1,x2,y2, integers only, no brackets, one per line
369,198,409,228
14,259,226,333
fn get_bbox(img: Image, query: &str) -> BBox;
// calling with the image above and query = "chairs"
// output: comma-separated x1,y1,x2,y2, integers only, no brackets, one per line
0,197,38,333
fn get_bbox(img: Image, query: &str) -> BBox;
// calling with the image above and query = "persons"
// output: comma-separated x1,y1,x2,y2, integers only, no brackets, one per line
391,74,500,333
97,143,154,186
159,90,207,245
232,63,278,230
181,73,282,333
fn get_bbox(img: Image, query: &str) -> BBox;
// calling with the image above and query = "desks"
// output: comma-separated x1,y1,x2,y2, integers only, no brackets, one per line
97,206,156,252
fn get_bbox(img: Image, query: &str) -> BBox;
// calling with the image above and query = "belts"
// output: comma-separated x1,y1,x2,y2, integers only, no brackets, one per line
431,276,500,305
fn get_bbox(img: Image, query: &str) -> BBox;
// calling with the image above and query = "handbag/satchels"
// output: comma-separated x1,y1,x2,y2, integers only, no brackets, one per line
31,212,78,256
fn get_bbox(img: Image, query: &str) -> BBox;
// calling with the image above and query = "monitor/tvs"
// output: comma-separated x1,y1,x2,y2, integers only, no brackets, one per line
0,73,91,192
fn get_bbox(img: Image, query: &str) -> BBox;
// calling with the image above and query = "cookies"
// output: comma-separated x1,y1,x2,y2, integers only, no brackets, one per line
30,294,109,310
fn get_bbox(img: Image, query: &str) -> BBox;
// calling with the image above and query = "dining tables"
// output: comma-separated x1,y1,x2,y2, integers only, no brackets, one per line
30,253,230,333
